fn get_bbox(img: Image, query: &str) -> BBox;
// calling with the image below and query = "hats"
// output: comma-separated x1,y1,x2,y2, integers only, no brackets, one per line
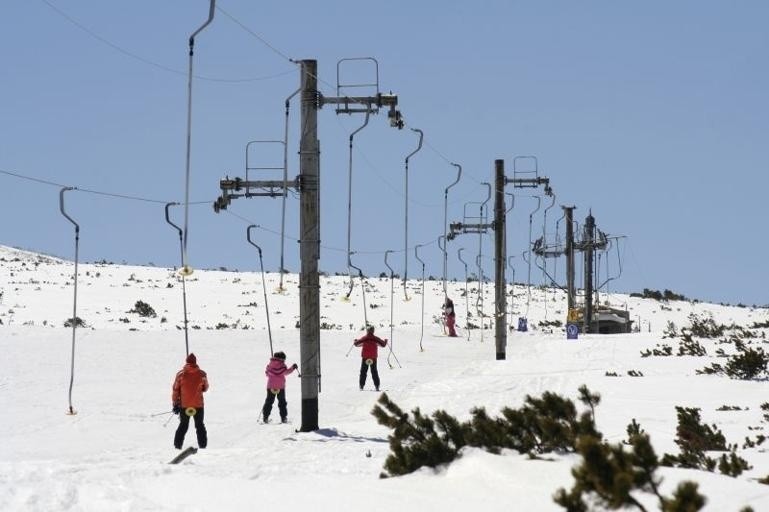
186,353,196,363
367,326,374,333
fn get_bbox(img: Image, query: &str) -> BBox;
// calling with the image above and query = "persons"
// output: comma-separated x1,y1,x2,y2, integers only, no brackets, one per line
442,298,460,338
353,326,388,392
171,353,209,449
262,352,299,424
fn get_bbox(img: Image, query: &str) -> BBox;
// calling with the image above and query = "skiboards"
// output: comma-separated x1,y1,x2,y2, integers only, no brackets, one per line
257,416,291,426
434,333,468,337
356,388,389,393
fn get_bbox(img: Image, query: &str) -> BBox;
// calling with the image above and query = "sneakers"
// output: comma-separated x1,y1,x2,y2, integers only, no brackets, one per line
360,384,379,390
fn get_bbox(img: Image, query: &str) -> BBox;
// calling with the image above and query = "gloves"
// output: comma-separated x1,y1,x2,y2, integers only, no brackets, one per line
293,364,297,369
174,404,181,414
